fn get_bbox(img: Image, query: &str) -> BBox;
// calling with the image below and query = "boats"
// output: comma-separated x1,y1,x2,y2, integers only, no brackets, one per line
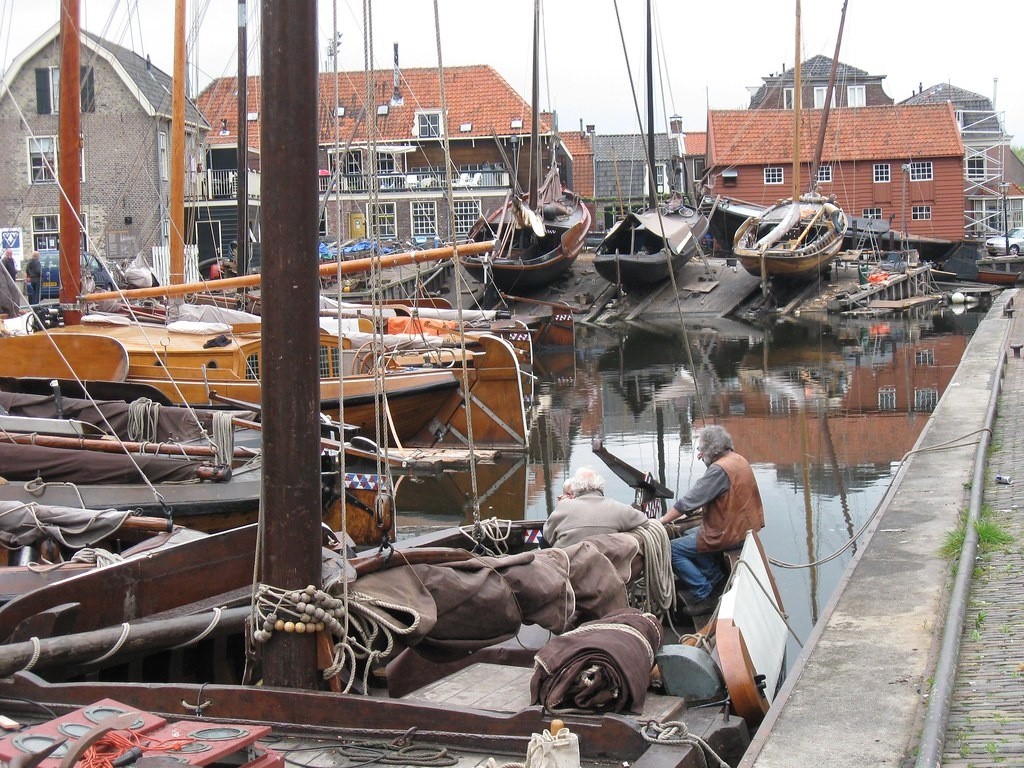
978,268,1021,286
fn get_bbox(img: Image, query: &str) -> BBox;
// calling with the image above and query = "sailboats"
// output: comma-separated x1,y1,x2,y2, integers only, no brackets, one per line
730,0,852,279
1,0,791,768
594,0,712,291
459,1,592,296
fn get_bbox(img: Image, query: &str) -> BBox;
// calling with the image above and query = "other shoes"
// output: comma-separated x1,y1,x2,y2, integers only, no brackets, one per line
694,576,732,604
682,592,719,616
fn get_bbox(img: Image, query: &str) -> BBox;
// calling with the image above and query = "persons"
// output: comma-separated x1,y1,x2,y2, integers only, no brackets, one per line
26,251,42,305
538,467,650,548
1,248,17,281
659,425,765,616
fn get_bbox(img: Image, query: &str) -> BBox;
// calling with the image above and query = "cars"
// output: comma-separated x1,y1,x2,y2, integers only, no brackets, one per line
987,226,1024,258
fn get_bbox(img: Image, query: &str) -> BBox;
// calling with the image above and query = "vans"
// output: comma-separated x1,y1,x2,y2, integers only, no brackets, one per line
24,248,115,307
